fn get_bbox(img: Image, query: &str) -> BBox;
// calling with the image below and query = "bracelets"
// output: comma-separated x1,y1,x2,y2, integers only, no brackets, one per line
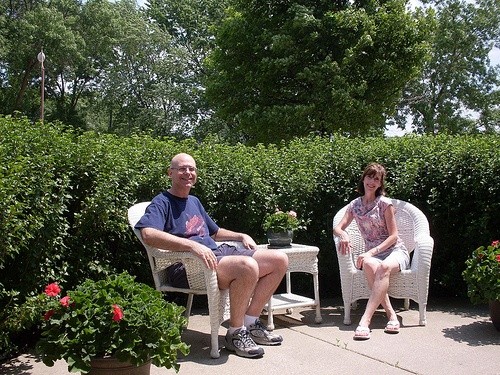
375,246,380,253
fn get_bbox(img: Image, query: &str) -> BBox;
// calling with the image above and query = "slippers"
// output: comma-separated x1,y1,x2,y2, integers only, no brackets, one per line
353,326,370,340
384,320,400,334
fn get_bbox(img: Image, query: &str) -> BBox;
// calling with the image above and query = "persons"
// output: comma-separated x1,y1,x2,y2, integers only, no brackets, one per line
333,162,410,340
133,153,289,358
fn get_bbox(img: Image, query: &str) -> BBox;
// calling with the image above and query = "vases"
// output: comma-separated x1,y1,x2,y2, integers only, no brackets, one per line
266,230,294,248
488,300,500,334
89,356,151,375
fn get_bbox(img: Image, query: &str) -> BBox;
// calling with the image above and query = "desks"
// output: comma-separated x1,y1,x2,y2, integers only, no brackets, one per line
257,243,323,331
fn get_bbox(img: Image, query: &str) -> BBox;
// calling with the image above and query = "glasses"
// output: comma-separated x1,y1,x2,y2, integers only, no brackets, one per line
171,167,199,173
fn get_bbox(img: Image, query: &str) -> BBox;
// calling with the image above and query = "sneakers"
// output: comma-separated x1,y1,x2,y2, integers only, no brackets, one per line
223,327,264,358
247,319,283,346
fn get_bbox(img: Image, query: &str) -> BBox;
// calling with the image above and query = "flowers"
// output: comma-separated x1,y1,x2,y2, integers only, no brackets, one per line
461,240,500,307
261,208,312,233
20,269,191,375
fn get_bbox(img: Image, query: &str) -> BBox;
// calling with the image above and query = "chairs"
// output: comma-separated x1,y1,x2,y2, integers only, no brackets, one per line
128,201,248,358
333,199,434,326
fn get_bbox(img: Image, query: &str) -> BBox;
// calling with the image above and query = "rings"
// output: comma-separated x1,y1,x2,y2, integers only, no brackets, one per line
206,257,211,261
